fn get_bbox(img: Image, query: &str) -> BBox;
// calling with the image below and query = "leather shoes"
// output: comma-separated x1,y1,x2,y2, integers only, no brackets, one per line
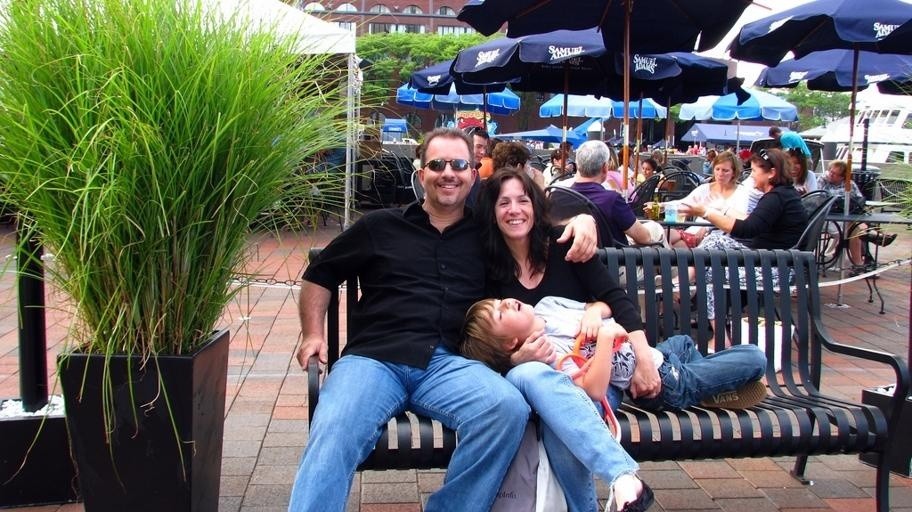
618,477,655,512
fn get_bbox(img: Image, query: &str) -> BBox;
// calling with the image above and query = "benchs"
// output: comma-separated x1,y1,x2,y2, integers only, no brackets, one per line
307,248,911,512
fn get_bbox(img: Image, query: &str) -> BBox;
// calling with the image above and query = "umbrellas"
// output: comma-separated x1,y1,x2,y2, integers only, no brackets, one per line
762,48,912,98
680,89,799,152
722,1,912,304
397,80,514,128
539,91,671,139
458,1,757,194
409,29,733,185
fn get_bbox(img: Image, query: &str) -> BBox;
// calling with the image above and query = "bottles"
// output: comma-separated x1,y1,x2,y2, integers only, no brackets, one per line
651,187,662,221
688,144,698,156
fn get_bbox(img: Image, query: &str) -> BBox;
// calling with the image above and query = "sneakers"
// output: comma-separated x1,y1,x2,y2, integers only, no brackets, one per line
882,233,898,248
698,378,768,411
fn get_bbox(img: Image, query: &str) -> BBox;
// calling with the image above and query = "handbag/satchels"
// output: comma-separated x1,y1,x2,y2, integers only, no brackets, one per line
729,313,796,377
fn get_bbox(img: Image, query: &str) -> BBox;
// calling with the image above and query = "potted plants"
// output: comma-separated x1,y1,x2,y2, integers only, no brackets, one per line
0,0,419,512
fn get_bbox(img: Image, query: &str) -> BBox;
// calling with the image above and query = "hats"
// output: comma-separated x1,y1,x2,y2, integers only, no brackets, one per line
551,148,570,160
739,148,752,160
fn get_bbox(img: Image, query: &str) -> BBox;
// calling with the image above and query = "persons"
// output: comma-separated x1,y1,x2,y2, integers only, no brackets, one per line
768,127,814,171
641,150,751,251
660,146,806,354
461,285,769,411
549,140,672,290
784,148,821,199
284,126,603,512
472,163,664,512
466,126,752,200
814,159,897,276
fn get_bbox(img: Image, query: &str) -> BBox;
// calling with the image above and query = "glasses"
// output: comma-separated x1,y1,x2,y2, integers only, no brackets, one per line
421,158,471,171
759,148,775,167
466,126,486,140
785,146,804,156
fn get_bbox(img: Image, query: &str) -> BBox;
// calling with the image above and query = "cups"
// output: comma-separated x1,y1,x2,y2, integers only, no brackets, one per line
674,213,686,224
664,204,678,224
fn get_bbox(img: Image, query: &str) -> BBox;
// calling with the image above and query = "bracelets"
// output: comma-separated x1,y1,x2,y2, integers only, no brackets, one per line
701,206,711,221
